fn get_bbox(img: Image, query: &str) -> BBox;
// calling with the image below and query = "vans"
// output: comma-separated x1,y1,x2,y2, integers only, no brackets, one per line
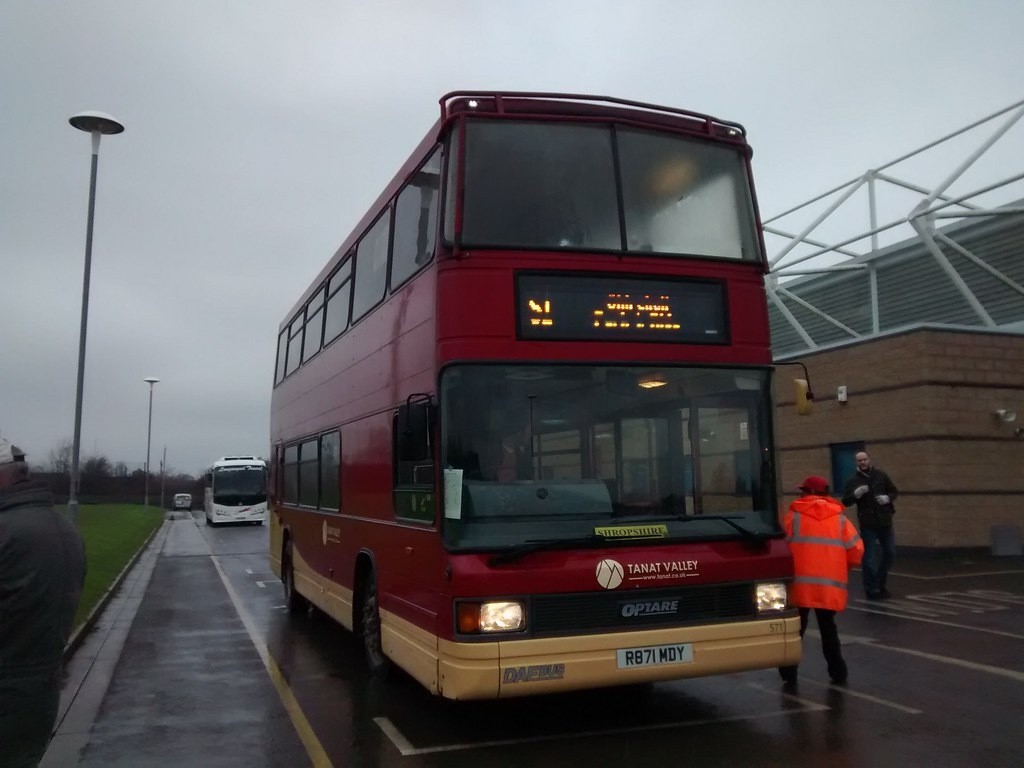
171,494,193,511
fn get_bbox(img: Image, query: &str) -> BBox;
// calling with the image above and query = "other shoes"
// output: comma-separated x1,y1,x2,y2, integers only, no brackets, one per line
878,587,892,600
865,590,884,600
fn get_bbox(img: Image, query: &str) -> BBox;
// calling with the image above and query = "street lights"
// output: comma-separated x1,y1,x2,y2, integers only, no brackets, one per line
58,111,129,518
144,376,160,507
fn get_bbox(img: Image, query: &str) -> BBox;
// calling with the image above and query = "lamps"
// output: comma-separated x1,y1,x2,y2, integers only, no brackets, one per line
995,409,1017,423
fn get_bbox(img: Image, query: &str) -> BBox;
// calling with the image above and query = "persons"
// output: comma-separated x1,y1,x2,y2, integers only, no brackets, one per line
778,476,865,685
842,447,899,600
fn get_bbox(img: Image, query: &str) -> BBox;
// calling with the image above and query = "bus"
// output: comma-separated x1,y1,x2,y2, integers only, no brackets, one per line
203,456,268,528
261,90,816,709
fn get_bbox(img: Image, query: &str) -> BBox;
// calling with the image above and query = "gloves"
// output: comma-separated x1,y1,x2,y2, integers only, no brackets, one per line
876,494,890,505
854,485,868,499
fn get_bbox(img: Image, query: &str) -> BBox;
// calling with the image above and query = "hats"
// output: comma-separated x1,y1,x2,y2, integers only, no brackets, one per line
796,476,829,495
